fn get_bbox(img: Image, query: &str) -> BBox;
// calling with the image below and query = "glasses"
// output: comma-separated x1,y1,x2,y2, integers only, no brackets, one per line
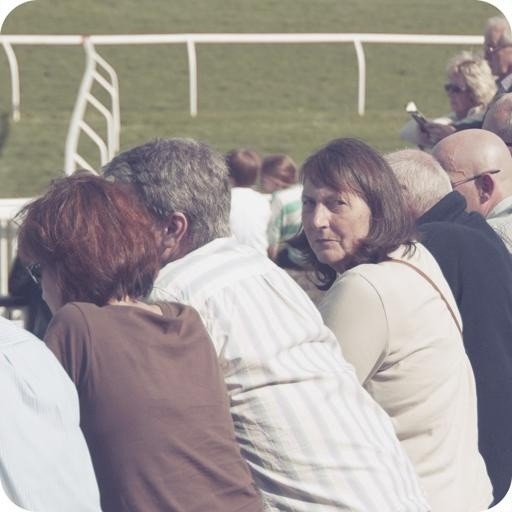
450,170,501,189
25,262,45,284
487,44,500,53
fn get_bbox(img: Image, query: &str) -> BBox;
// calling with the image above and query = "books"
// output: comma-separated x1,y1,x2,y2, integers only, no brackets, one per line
405,101,430,128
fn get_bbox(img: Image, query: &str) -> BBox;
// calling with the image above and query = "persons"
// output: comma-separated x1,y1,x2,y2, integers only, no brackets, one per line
1,313,102,512
106,135,432,511
222,146,272,260
436,127,512,256
11,168,264,511
282,139,495,512
383,146,512,512
261,151,312,269
484,15,512,94
400,51,497,145
480,90,512,147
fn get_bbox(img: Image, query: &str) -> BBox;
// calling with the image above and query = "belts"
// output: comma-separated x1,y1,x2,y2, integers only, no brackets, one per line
444,84,462,94
